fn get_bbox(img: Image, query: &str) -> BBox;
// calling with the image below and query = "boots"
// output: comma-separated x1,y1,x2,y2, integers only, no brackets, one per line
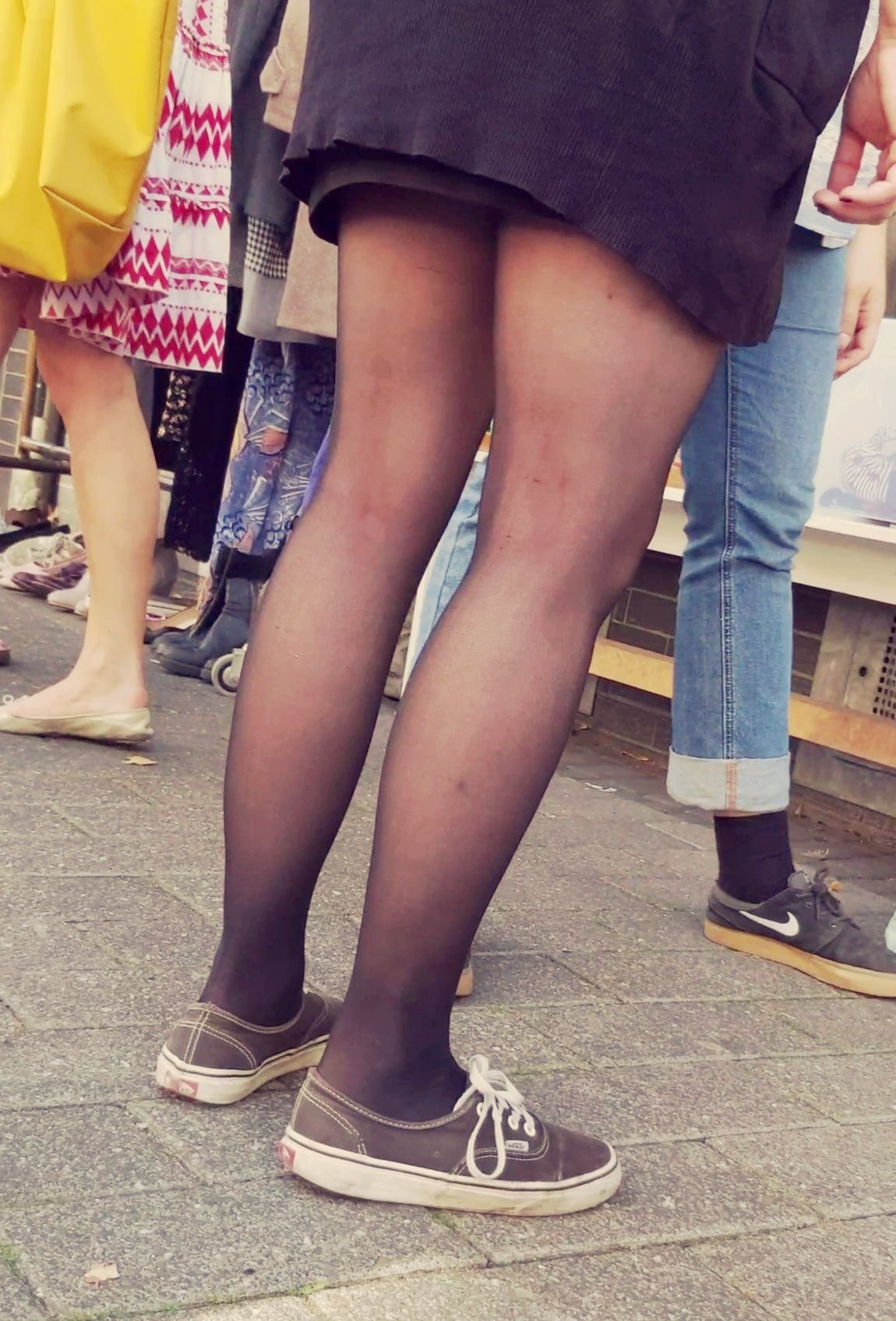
150,578,258,683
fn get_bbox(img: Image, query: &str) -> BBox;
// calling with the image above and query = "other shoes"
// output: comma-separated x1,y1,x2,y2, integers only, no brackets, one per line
0,691,154,743
702,870,895,1000
278,1052,625,1215
11,560,90,616
156,983,342,1104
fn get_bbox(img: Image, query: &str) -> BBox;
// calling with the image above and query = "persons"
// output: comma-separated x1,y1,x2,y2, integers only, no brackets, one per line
1,0,896,1217
157,1,896,1216
